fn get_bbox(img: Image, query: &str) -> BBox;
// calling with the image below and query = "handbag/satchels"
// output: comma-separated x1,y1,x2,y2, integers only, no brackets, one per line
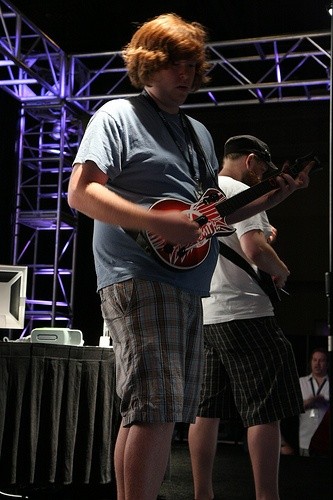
257,268,281,306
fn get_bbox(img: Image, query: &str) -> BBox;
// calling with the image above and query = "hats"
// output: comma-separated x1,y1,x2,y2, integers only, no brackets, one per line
224,135,278,170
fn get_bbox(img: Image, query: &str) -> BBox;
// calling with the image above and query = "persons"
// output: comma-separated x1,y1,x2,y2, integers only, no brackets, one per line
186,135,295,500
296,347,333,500
68,11,318,500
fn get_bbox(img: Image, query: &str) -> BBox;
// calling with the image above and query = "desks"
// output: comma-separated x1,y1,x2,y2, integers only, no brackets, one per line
0,341,115,488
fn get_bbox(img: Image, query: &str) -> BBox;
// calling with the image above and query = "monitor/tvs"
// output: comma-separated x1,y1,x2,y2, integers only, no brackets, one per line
0,265,28,329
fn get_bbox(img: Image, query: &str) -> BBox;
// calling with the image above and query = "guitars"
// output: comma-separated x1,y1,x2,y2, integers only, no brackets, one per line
144,147,329,274
